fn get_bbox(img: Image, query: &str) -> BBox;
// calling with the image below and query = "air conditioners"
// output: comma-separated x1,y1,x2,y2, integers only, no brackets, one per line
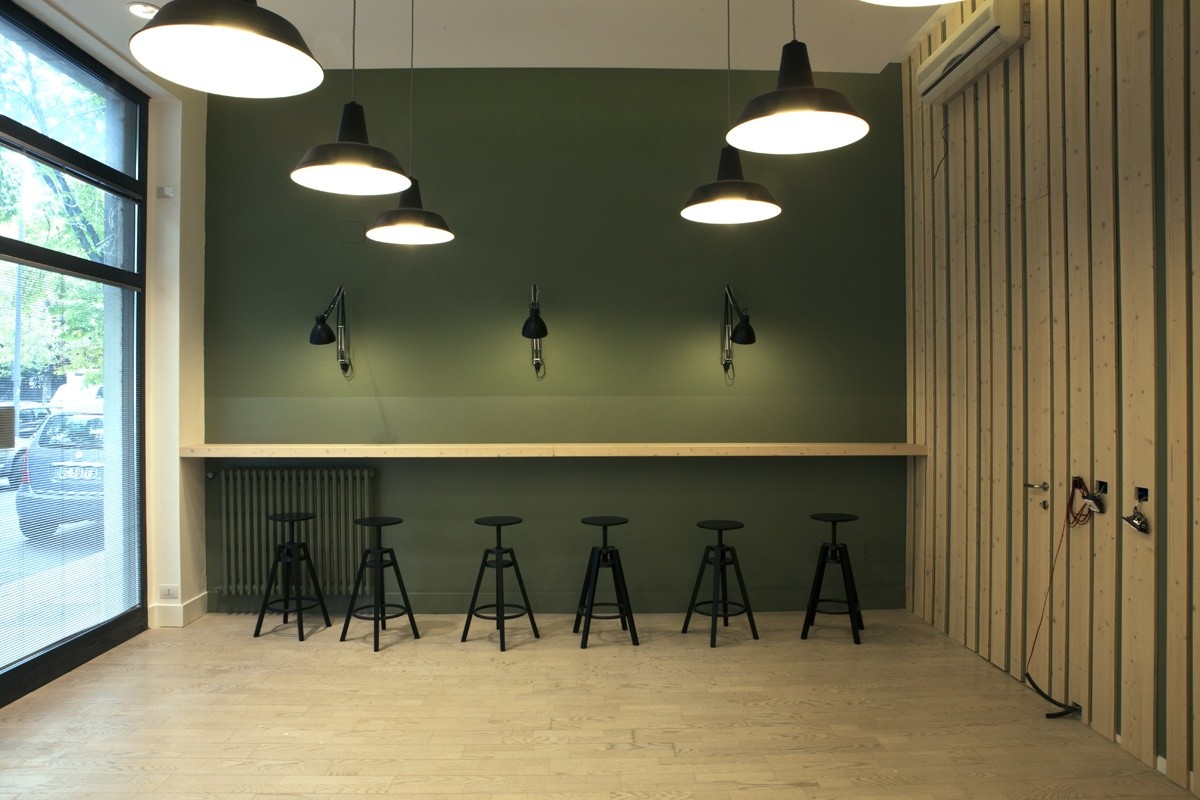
913,0,1030,105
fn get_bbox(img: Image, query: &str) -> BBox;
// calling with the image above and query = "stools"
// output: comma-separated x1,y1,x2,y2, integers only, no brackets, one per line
341,516,421,652
682,520,759,649
801,512,866,645
254,510,331,642
460,515,542,650
572,516,641,649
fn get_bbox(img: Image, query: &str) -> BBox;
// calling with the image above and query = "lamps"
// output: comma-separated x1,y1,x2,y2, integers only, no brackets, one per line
721,289,756,384
310,284,355,378
679,1,782,224
520,282,546,380
726,0,870,156
128,0,325,99
291,0,411,198
365,1,454,246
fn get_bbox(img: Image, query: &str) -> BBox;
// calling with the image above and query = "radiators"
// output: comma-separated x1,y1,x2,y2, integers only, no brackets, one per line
205,467,375,598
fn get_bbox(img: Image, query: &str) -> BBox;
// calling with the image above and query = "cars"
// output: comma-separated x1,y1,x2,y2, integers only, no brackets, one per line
16,413,104,540
0,401,65,489
